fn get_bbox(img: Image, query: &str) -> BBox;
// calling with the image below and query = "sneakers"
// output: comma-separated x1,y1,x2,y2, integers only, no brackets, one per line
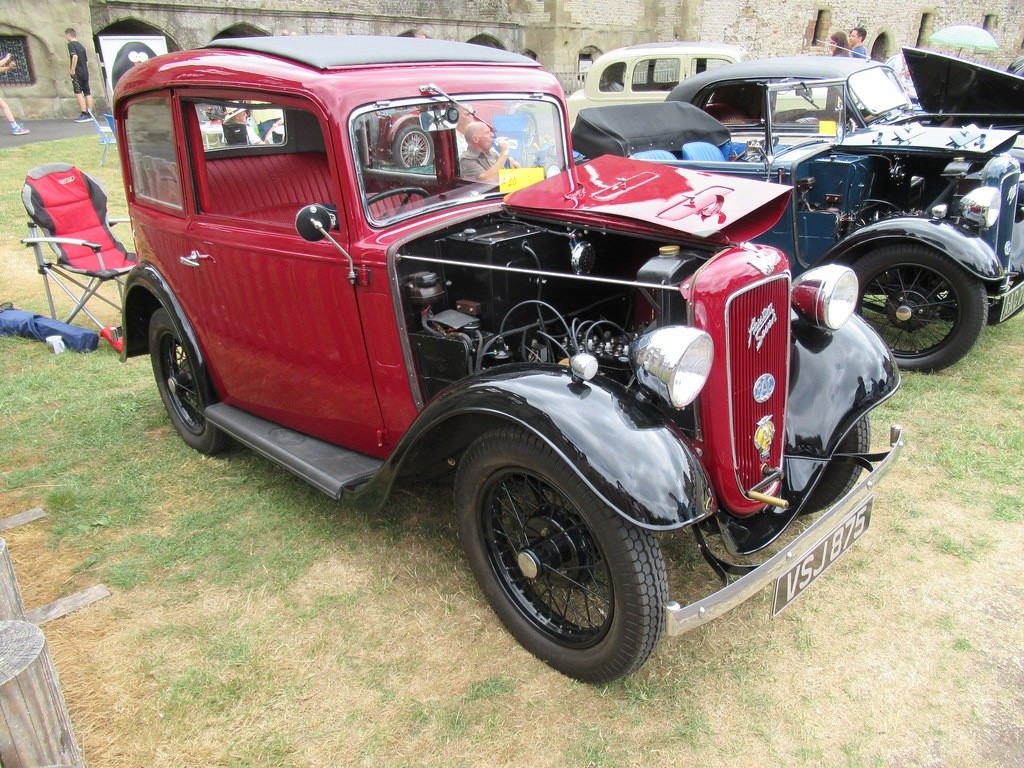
74,112,93,122
12,125,30,135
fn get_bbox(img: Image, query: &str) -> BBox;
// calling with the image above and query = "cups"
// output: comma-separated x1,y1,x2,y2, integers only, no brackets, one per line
46,335,65,354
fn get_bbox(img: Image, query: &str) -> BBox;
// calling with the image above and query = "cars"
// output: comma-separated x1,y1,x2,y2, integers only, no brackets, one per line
563,56,1023,375
117,34,908,686
559,44,759,140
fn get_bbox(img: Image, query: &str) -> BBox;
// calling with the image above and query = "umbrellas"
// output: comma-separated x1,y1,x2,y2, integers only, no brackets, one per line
929,25,999,58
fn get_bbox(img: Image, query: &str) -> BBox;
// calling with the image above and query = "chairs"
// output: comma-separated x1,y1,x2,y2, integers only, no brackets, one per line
631,149,677,161
104,113,114,135
17,162,139,354
87,109,117,166
221,123,252,146
681,142,726,162
271,131,283,144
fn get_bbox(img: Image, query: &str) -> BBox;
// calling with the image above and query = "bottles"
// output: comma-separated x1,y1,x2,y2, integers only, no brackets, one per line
492,138,518,149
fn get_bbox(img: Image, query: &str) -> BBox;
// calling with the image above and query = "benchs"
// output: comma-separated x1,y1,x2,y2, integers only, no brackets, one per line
143,151,427,234
796,117,819,124
703,103,750,124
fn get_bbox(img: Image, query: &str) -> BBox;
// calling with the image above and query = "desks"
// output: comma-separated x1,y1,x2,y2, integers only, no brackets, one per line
199,120,227,150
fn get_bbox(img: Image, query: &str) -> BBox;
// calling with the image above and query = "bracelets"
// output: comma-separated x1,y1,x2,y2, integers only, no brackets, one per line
273,124,279,127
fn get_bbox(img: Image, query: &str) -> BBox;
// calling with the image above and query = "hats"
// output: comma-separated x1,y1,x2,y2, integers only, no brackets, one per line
224,101,247,121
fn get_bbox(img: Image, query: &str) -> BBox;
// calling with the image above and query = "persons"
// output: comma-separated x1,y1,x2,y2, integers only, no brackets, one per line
459,122,522,184
0,52,30,135
223,100,285,146
885,52,919,104
65,28,95,123
456,103,474,159
826,27,866,110
